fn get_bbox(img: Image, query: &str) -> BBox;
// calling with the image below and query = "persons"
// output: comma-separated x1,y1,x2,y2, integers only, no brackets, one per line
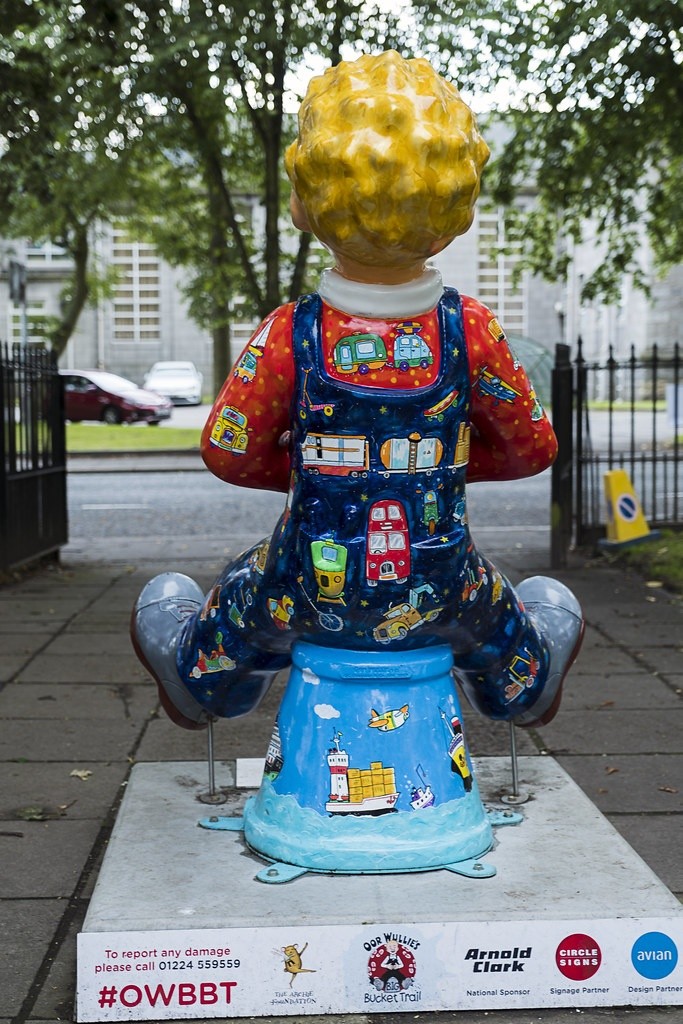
129,50,586,728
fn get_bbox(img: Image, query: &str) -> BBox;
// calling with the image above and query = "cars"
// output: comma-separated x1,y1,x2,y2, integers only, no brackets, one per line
41,369,174,426
141,361,205,406
